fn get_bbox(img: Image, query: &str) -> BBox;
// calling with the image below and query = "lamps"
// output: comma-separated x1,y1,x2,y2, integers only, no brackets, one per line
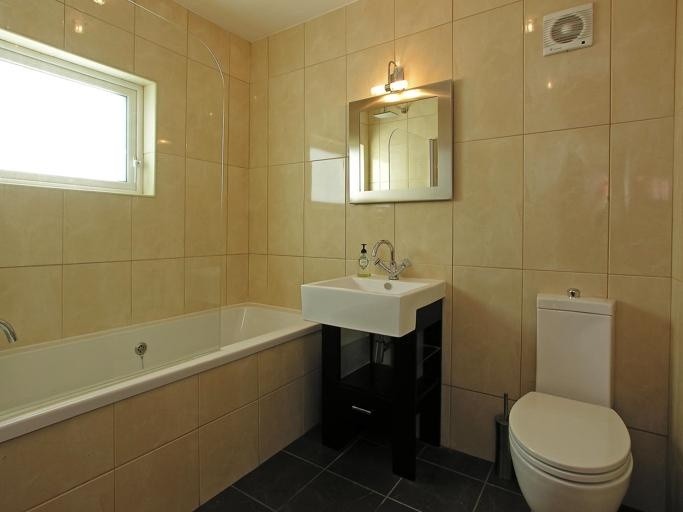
370,61,408,96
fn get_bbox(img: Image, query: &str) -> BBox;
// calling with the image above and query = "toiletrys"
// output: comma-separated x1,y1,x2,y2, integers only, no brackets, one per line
357,244,370,277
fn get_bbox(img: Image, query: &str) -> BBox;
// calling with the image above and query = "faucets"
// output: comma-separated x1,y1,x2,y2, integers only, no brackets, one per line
370,238,412,280
0,319,18,344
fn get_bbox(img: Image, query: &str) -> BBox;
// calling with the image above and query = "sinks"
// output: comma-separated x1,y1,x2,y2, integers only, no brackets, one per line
302,274,447,337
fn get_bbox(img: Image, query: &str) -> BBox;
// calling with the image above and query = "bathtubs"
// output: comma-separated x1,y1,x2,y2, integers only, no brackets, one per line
0,300,371,512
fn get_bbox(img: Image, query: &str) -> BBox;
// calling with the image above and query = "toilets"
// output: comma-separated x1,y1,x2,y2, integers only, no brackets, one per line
508,293,634,512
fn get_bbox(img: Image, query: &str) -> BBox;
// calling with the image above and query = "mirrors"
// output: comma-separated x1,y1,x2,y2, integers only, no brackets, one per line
349,79,453,204
